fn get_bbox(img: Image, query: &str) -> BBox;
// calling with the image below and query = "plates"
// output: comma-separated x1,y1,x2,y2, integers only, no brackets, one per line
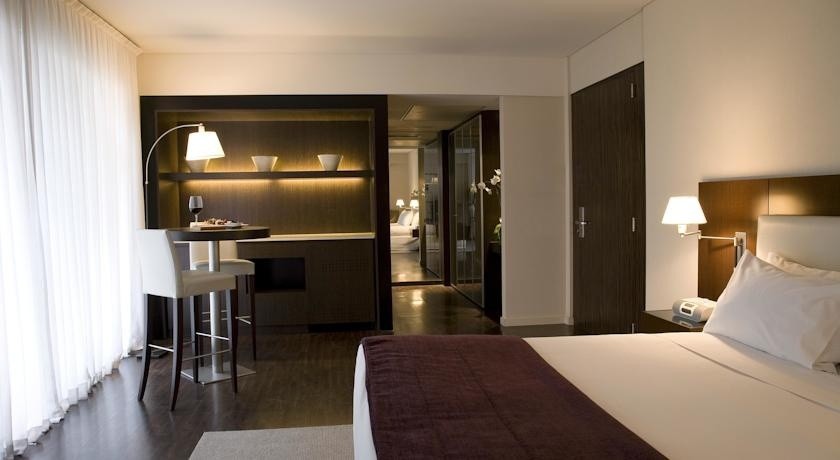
199,222,248,228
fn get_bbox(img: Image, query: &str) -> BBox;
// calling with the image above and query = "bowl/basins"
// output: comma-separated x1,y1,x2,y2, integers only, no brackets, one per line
184,156,209,172
250,156,279,172
317,154,344,172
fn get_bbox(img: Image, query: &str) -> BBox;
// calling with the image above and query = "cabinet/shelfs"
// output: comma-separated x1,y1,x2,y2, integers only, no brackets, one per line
157,112,378,330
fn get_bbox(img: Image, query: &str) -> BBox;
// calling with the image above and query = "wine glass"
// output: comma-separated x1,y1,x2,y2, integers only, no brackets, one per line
189,195,203,222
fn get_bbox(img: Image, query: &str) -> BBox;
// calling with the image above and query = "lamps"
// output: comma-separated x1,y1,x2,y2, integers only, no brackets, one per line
661,195,742,247
409,200,419,211
129,121,226,358
395,199,412,209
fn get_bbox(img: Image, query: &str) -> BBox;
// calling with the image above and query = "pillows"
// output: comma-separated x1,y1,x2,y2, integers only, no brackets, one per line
765,251,840,371
701,249,840,369
398,210,412,226
410,211,419,226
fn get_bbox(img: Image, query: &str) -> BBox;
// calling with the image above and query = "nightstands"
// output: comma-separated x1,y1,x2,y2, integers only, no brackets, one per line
638,309,707,334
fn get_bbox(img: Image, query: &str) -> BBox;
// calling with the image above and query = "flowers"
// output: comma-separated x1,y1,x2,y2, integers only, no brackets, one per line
477,167,502,242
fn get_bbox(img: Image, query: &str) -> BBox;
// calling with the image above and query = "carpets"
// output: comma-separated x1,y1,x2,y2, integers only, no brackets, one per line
190,425,353,460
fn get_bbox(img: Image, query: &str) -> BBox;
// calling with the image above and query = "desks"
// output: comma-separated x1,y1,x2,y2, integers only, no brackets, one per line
165,223,270,384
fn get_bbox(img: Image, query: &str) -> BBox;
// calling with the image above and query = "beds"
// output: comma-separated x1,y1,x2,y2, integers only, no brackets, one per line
352,213,840,460
389,211,422,253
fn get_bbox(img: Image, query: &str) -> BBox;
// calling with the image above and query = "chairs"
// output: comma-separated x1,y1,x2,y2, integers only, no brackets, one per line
188,241,259,367
133,229,239,412
